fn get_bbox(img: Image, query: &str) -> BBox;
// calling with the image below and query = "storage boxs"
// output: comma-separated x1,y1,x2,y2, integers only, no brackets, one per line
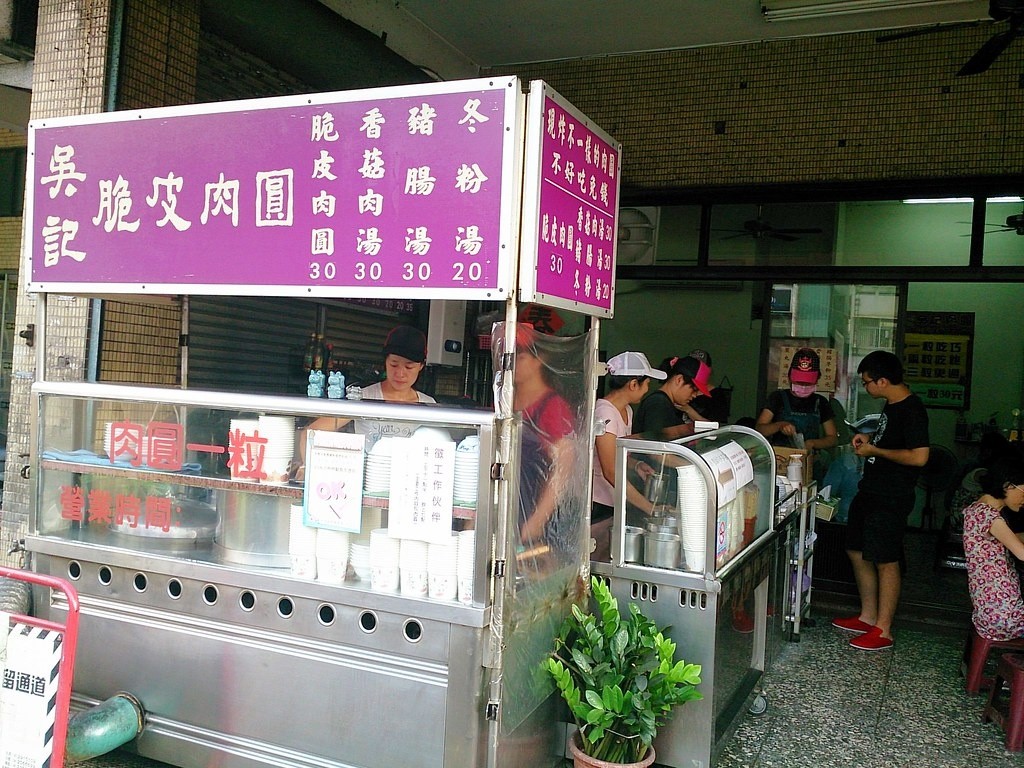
771,445,814,482
812,494,840,522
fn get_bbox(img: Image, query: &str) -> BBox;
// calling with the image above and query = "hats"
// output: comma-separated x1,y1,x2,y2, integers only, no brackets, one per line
790,350,820,382
607,351,667,380
517,322,536,355
676,356,712,397
382,326,427,362
688,349,712,367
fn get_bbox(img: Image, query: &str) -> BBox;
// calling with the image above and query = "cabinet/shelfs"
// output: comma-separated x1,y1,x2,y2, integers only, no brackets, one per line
30,382,496,605
790,479,818,644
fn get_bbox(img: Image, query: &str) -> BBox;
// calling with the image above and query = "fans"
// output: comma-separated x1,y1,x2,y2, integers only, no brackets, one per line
695,205,823,243
955,202,1024,237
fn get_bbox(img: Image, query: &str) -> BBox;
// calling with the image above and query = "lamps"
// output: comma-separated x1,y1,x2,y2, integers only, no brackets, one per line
762,0,968,23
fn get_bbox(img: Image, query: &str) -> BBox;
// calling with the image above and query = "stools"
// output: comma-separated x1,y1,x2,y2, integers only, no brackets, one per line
982,655,1023,756
957,626,1023,692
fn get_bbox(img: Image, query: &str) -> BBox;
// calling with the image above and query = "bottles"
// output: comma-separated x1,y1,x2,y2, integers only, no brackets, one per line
304,332,335,372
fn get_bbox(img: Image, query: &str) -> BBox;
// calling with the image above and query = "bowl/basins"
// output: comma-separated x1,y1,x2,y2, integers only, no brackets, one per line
754,455,803,512
289,502,496,604
103,423,177,465
675,465,745,572
227,415,295,485
643,533,682,570
652,511,680,518
362,425,499,502
641,516,681,533
609,525,645,562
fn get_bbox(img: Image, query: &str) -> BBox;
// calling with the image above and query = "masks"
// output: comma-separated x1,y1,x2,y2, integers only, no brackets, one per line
791,383,816,397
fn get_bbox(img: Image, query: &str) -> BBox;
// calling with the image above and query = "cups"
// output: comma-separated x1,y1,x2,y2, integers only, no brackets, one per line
644,474,672,503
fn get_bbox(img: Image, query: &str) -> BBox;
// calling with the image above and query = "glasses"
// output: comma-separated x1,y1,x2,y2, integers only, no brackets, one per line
861,378,881,387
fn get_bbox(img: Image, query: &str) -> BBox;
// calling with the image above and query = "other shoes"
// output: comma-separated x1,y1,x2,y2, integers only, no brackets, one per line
832,614,871,633
732,611,753,633
849,625,894,650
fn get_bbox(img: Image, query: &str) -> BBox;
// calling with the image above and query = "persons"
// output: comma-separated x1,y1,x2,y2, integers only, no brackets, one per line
948,443,1024,690
733,573,777,633
831,350,930,651
590,345,838,528
293,324,438,487
495,323,582,563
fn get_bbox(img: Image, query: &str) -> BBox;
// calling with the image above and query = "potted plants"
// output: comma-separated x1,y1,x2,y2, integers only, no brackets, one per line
543,575,703,768
